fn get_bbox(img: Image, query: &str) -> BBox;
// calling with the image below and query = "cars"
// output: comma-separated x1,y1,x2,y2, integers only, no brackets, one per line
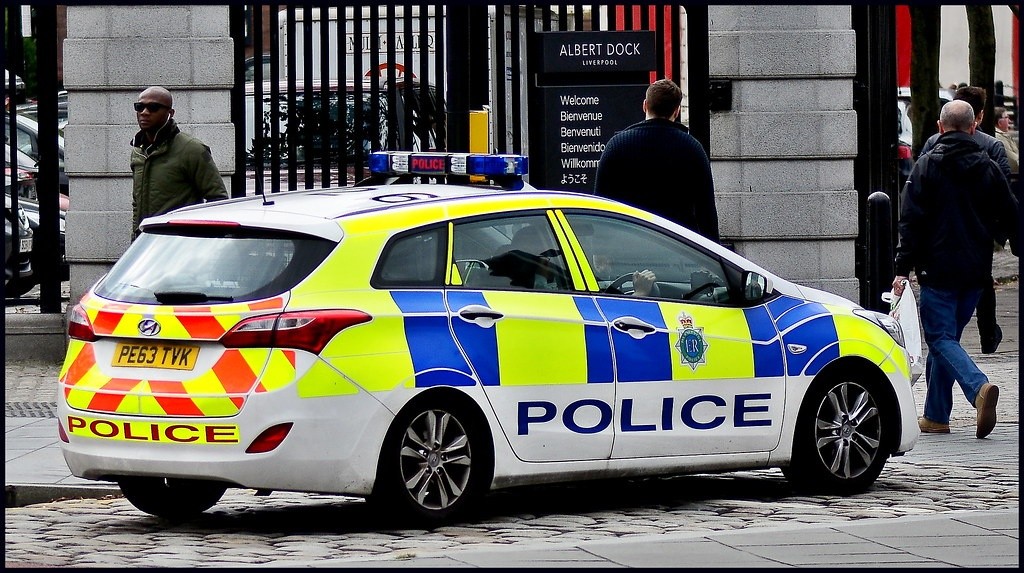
240,80,446,196
5,92,69,299
58,150,921,524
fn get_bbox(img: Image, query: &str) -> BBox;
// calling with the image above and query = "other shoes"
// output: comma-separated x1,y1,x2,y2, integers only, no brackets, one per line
980,324,1002,354
994,242,1003,253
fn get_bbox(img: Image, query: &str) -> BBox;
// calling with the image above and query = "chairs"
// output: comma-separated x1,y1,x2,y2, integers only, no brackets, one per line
489,243,537,289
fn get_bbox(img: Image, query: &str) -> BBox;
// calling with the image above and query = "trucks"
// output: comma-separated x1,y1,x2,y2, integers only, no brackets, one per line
276,5,575,184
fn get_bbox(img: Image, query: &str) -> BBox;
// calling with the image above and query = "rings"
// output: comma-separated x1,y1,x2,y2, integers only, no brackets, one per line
896,288,899,291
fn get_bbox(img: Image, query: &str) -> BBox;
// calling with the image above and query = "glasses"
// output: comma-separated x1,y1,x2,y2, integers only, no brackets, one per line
134,102,171,113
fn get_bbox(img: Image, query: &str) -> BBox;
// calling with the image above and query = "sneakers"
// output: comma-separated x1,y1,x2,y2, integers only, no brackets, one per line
917,417,950,433
975,383,999,439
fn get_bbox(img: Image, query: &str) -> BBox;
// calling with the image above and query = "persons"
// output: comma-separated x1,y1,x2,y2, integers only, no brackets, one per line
510,226,657,296
594,78,719,273
130,86,229,244
892,83,1019,439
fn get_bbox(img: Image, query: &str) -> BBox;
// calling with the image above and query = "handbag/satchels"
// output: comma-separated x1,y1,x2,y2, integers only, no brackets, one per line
881,280,923,386
